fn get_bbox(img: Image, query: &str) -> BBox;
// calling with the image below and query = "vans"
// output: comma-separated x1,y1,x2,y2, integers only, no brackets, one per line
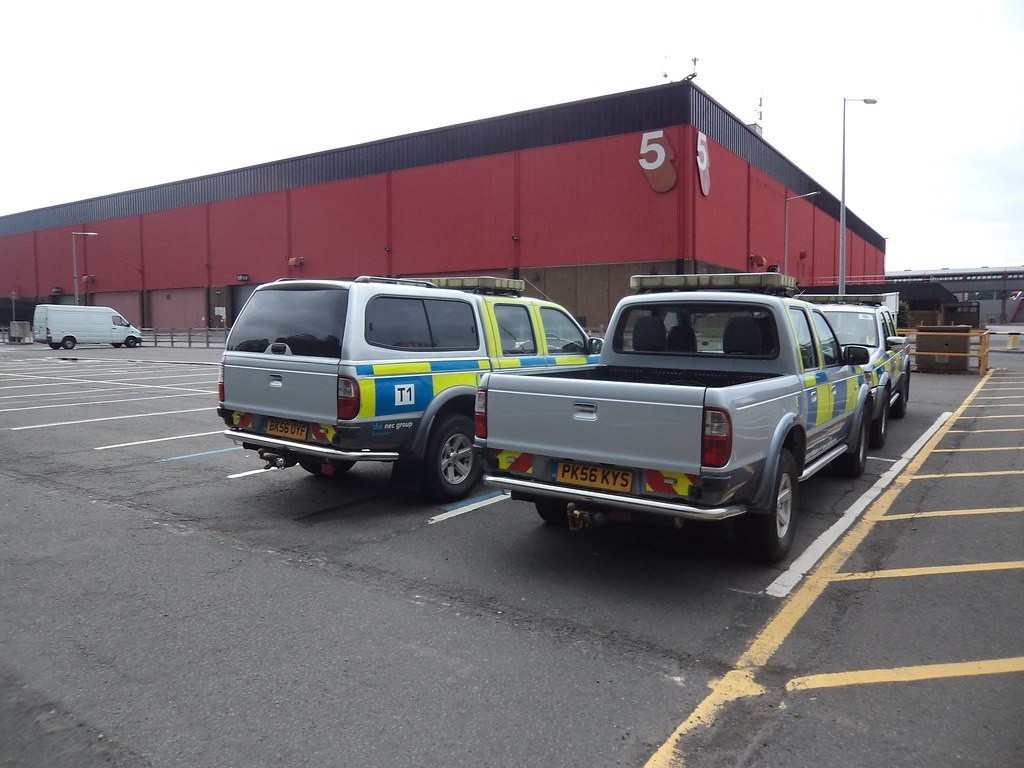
32,303,144,351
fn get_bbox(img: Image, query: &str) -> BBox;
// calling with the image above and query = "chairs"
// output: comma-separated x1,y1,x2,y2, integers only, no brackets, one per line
723,316,762,354
632,316,666,351
666,325,698,353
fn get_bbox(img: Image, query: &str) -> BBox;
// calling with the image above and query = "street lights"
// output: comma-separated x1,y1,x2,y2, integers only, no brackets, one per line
71,231,99,306
837,99,880,294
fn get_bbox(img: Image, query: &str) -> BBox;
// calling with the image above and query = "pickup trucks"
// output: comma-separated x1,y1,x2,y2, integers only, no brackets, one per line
795,292,911,450
473,272,872,564
218,274,604,501
